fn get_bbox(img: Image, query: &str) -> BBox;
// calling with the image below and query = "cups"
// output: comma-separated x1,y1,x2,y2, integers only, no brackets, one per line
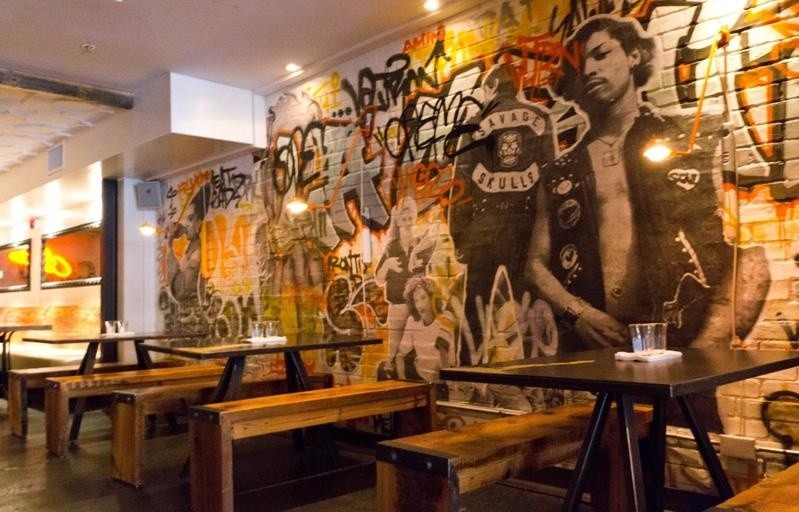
251,321,279,339
628,322,668,356
104,320,129,335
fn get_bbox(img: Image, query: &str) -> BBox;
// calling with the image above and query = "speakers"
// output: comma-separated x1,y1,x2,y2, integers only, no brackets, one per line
135,182,162,210
47,142,66,175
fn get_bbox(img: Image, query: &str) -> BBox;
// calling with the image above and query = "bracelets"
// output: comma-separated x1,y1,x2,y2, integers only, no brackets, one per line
558,295,593,334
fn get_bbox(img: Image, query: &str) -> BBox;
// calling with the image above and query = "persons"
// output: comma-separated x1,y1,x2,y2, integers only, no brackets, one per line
372,196,440,359
525,13,772,435
449,63,556,366
164,182,211,307
395,275,454,387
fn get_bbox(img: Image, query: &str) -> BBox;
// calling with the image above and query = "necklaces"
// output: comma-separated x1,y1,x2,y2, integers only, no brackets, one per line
589,126,631,168
